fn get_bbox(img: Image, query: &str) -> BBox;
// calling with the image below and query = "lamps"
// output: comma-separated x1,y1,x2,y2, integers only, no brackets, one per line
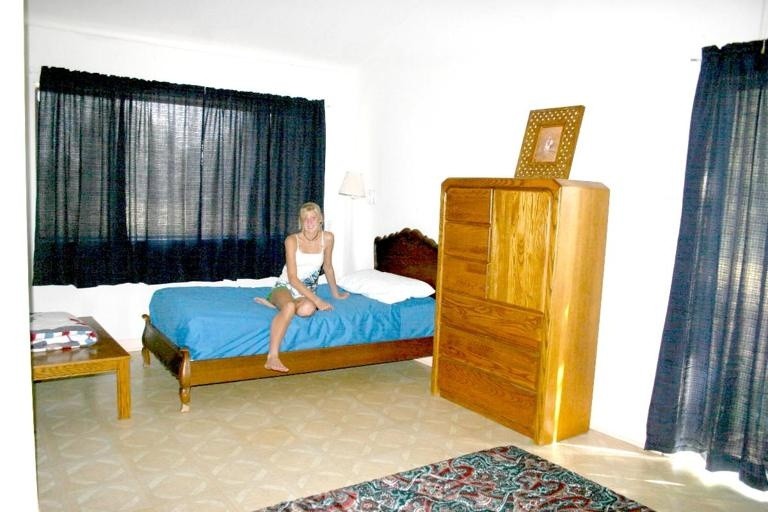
337,170,366,270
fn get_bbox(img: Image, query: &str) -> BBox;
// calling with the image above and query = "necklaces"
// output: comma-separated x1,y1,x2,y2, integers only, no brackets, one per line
302,229,321,241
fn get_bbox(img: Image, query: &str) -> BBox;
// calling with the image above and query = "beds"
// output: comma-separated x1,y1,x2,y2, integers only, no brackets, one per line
140,227,438,414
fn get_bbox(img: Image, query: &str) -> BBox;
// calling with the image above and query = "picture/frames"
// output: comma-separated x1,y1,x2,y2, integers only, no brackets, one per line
513,103,585,180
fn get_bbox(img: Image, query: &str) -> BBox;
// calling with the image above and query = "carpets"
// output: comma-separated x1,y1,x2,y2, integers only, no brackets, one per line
253,443,657,512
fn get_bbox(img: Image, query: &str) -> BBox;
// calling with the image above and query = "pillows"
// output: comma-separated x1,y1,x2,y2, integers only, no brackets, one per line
330,266,434,305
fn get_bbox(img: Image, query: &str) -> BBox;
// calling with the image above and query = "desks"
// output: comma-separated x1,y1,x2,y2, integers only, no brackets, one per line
32,315,133,421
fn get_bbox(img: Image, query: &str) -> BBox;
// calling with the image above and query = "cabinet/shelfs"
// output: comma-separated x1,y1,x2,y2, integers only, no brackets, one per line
427,174,611,447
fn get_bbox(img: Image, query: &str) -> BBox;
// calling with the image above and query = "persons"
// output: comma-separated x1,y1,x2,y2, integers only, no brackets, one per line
253,203,349,373
